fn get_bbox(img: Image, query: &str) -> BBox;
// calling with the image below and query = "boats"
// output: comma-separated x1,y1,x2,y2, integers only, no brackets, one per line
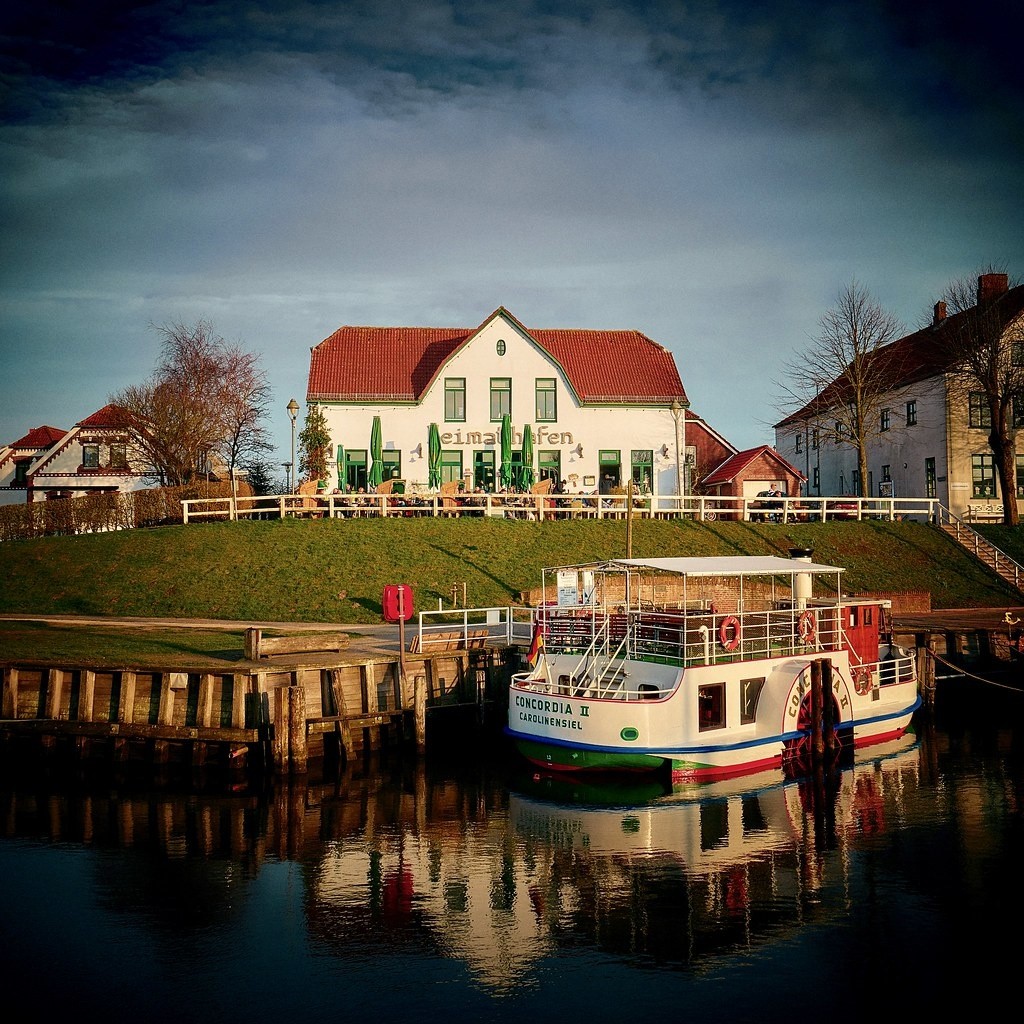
504,556,926,788
505,726,928,942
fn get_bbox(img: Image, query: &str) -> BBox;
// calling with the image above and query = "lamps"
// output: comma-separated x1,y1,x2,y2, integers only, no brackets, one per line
578,443,584,463
418,443,423,462
662,444,670,461
327,443,334,459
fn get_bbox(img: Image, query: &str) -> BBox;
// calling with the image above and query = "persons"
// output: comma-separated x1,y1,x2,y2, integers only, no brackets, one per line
319,473,654,520
767,483,801,523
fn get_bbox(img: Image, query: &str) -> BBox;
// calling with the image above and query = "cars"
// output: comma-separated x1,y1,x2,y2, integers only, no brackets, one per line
826,494,870,520
750,491,799,524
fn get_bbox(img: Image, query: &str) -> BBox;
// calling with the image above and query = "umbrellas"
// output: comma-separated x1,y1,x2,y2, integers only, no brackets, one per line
369,415,384,490
428,423,442,495
500,413,514,505
521,425,536,502
337,444,347,492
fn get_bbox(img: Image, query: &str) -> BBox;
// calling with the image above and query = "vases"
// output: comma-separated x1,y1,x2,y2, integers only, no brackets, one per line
638,502,646,508
563,503,572,508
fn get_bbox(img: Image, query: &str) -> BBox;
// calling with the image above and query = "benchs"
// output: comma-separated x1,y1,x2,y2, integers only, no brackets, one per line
967,504,1004,523
748,494,869,525
546,607,801,669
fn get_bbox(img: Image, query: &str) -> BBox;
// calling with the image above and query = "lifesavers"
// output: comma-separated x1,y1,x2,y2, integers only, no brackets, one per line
798,611,816,641
576,610,592,621
720,616,741,650
855,668,872,695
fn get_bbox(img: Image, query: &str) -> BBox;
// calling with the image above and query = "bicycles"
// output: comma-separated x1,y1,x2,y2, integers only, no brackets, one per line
704,505,717,521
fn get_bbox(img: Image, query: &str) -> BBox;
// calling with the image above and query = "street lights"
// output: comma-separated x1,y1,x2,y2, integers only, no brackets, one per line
281,461,293,505
668,397,686,520
286,399,301,519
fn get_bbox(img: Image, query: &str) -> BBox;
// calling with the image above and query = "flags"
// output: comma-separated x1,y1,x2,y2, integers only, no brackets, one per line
527,620,543,667
